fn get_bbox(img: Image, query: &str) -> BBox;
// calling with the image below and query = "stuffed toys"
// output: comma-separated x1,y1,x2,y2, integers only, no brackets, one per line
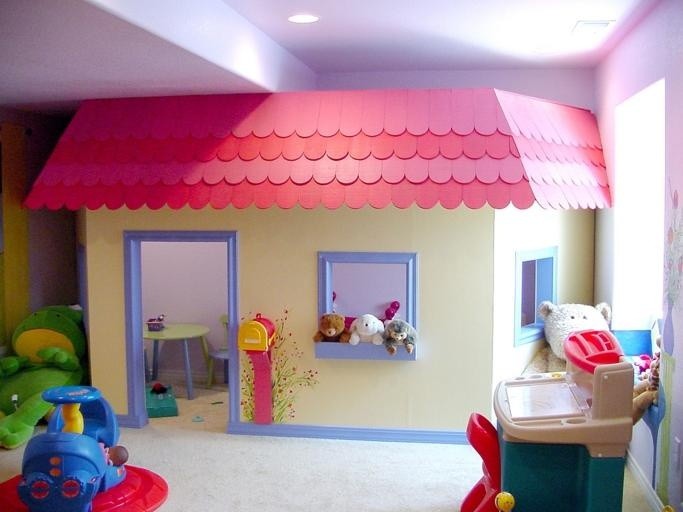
313,314,416,355
0,305,86,450
522,300,612,373
633,353,660,426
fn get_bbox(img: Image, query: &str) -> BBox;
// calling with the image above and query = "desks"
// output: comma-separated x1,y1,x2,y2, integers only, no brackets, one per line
142,323,217,400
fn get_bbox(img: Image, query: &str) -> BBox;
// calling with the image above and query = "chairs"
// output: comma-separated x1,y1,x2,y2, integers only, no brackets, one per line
204,314,228,389
458,412,500,511
